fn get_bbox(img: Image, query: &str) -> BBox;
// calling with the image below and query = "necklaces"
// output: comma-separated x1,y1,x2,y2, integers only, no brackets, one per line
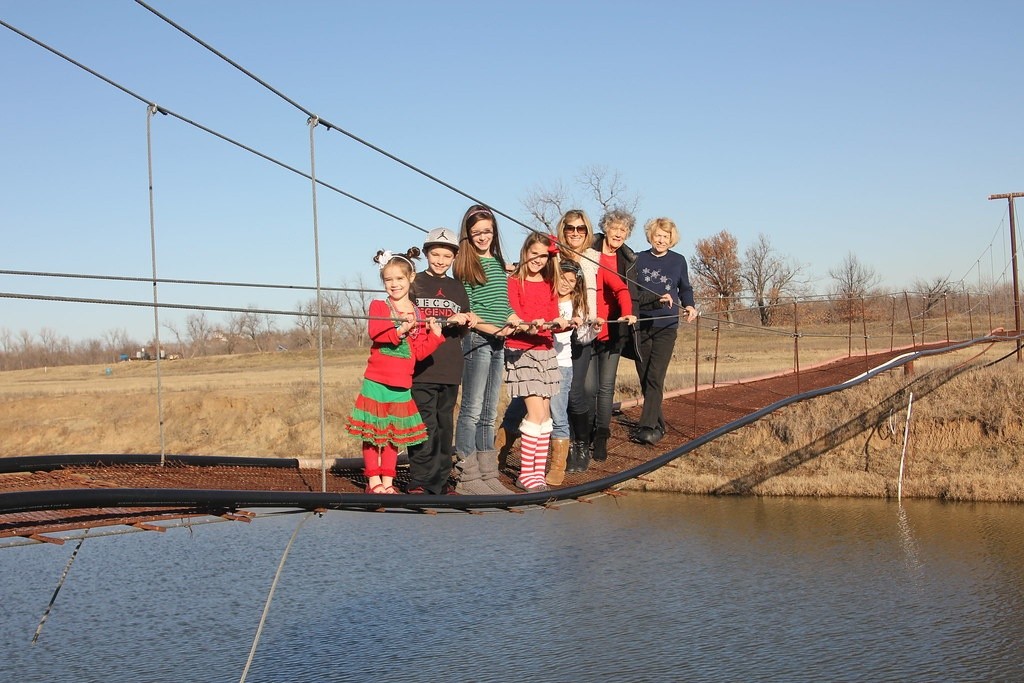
386,296,421,339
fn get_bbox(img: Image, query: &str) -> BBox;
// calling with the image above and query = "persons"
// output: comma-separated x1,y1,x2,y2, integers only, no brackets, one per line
452,204,538,495
632,218,699,445
508,231,582,494
503,257,604,487
408,228,478,498
556,210,637,474
344,248,429,494
589,209,672,462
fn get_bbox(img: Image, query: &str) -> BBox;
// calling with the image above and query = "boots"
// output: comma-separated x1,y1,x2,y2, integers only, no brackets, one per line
546,437,570,486
593,427,610,462
477,450,516,495
564,409,592,473
454,449,497,495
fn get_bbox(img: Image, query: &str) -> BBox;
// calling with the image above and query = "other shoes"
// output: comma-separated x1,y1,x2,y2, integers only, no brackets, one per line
365,484,402,494
516,478,551,492
408,485,432,495
439,484,458,496
639,422,666,443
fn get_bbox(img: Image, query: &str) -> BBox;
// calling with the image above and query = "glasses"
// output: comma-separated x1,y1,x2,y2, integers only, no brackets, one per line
564,226,587,234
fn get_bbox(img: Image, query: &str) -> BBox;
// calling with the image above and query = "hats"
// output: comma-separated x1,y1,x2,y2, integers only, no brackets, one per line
424,227,460,250
